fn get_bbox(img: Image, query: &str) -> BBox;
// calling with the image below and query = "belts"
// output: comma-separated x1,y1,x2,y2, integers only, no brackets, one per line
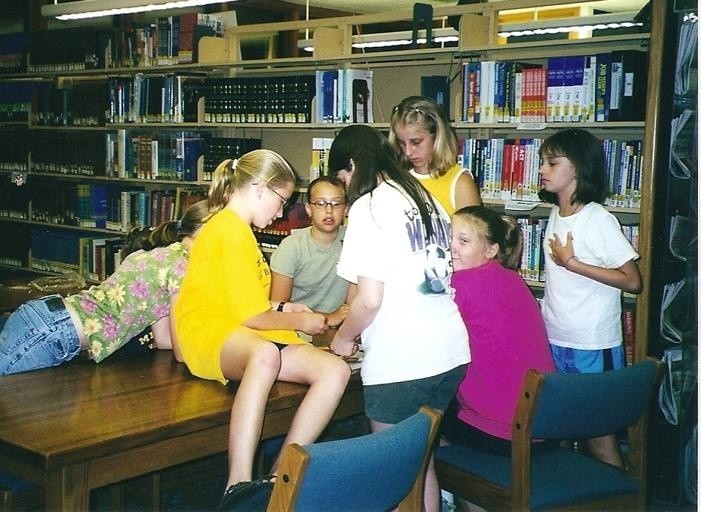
46,294,79,352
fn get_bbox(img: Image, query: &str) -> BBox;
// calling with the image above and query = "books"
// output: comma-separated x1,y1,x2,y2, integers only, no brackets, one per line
0,69,373,123
1,13,222,73
451,48,648,368
2,173,206,284
1,131,261,182
250,138,336,249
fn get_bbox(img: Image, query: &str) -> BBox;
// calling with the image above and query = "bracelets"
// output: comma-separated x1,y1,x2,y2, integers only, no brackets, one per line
277,302,286,313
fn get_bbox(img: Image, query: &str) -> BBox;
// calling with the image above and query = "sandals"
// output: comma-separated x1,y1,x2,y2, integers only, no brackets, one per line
216,474,277,512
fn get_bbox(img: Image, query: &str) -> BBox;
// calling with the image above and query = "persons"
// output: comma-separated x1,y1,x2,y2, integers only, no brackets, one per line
0,201,211,377
536,128,642,472
328,95,556,512
174,149,351,512
271,176,357,326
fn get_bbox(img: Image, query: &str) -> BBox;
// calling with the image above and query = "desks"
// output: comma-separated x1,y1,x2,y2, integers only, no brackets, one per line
0,347,365,510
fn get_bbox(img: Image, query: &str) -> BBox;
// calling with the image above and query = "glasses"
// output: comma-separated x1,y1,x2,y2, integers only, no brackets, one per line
310,199,346,209
253,182,290,208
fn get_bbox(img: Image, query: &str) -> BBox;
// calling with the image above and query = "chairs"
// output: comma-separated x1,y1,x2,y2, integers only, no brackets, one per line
267,405,442,511
428,357,663,510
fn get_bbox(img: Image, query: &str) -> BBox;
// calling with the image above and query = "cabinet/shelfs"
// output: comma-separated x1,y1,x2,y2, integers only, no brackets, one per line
1,0,667,462
